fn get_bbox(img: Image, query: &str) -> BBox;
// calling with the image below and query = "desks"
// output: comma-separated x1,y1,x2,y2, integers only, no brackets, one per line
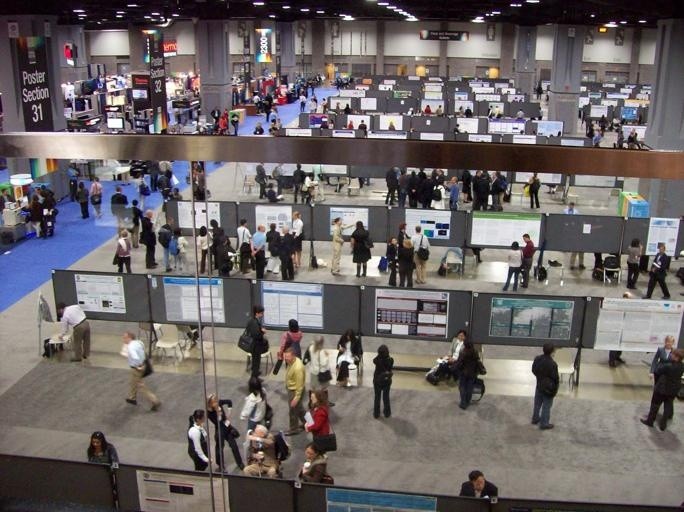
94,163,130,182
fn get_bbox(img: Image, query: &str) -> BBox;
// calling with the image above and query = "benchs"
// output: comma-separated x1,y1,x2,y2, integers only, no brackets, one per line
135,321,203,351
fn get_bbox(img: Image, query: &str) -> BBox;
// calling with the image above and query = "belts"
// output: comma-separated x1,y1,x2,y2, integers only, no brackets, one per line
73,318,87,328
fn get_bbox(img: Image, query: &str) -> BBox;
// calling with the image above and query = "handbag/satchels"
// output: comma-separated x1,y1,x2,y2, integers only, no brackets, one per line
477,359,486,376
417,247,429,260
91,195,101,204
143,357,153,378
319,371,332,382
312,435,337,452
237,330,255,353
228,424,240,438
241,242,251,256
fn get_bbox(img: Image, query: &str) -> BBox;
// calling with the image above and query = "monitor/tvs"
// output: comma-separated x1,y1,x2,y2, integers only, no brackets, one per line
132,87,149,102
107,117,124,134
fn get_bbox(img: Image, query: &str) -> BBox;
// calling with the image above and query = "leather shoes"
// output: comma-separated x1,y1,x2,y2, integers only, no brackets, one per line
151,402,161,412
238,462,244,470
284,429,300,436
126,399,137,405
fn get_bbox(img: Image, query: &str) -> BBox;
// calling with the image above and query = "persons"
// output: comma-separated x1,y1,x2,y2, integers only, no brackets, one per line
608,291,634,369
1,158,208,274
372,344,395,419
64,69,658,151
330,165,541,292
86,432,119,466
119,331,161,411
57,302,92,362
563,202,586,270
460,469,498,498
426,330,486,410
187,304,363,487
531,341,561,430
629,238,671,300
640,334,684,432
193,162,325,283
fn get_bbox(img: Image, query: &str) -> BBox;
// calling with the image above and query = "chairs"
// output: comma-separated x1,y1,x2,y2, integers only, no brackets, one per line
335,351,363,378
239,172,260,194
244,344,274,375
602,254,621,285
153,323,184,367
44,320,75,362
445,257,463,279
347,176,364,197
506,184,625,209
544,257,566,287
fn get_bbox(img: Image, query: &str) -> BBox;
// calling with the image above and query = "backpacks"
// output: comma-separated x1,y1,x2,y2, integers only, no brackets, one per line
169,237,178,254
378,369,393,388
